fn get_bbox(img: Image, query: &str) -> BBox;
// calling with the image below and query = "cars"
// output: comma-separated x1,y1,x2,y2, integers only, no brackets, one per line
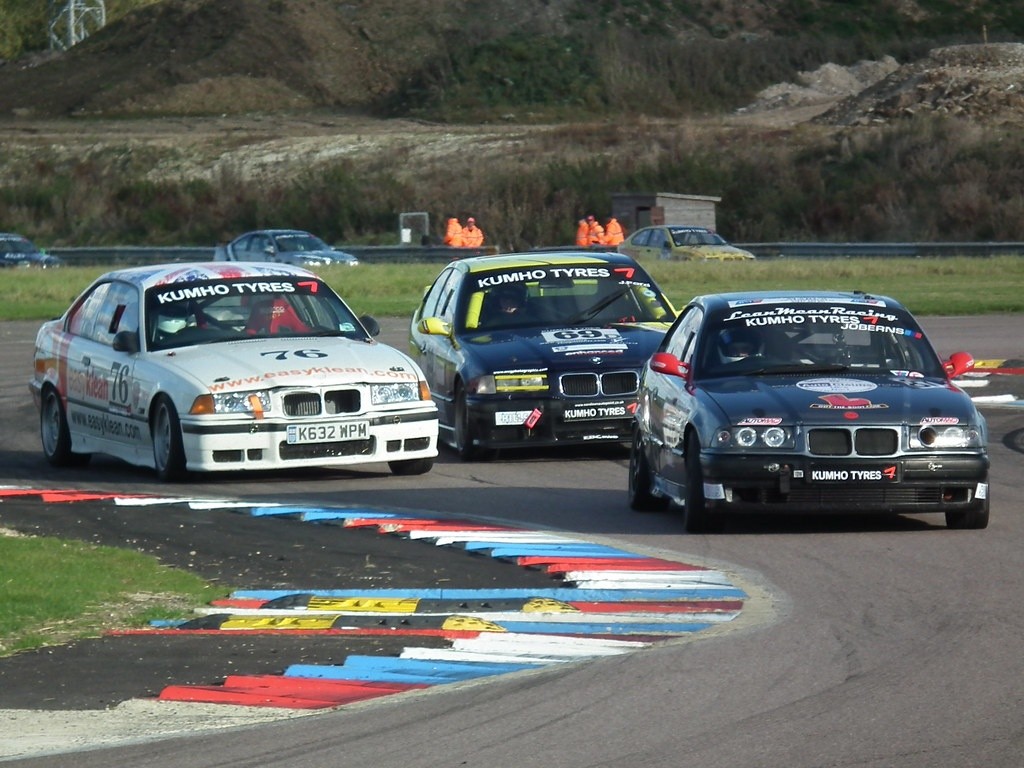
212,228,360,266
0,231,66,269
618,223,757,263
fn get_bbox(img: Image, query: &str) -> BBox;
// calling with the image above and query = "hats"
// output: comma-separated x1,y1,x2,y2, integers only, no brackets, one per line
588,215,595,220
467,218,475,222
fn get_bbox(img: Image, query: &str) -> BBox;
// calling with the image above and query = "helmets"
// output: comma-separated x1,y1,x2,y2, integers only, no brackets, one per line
490,282,529,306
156,300,188,333
717,326,766,364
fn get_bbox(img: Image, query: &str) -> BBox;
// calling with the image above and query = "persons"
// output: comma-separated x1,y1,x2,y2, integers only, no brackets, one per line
442,213,484,247
716,323,772,367
483,278,537,326
576,214,625,246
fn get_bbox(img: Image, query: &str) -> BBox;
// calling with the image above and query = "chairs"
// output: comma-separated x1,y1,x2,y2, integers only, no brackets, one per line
247,299,311,333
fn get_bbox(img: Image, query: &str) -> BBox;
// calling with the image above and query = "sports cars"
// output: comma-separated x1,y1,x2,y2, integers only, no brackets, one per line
625,287,990,534
29,261,440,483
407,247,682,462
